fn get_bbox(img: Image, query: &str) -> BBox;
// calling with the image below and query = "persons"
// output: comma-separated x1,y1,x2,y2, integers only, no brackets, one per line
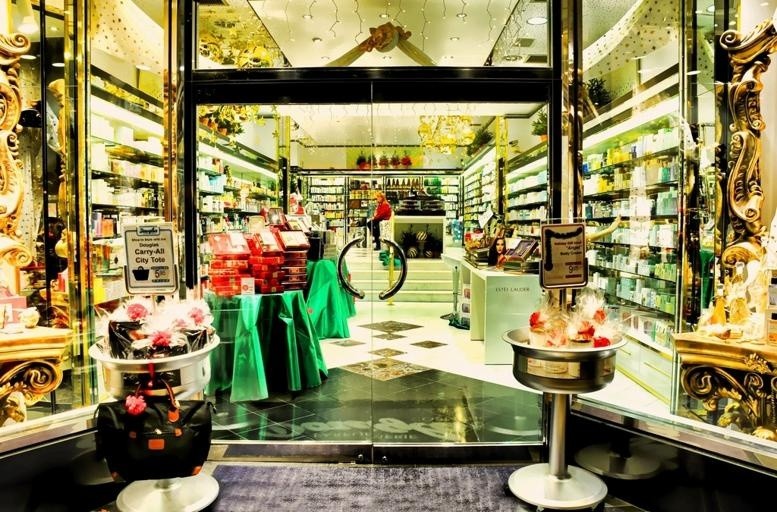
487,237,506,266
586,214,623,242
366,192,391,252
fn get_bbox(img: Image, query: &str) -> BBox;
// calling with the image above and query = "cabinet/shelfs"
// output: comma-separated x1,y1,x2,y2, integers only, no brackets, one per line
300,170,463,253
90,51,308,346
462,63,680,400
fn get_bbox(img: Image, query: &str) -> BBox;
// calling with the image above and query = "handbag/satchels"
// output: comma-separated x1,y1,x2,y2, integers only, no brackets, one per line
94,376,217,483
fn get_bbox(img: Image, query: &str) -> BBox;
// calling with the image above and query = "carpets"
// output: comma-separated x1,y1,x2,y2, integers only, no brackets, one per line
204,463,548,510
